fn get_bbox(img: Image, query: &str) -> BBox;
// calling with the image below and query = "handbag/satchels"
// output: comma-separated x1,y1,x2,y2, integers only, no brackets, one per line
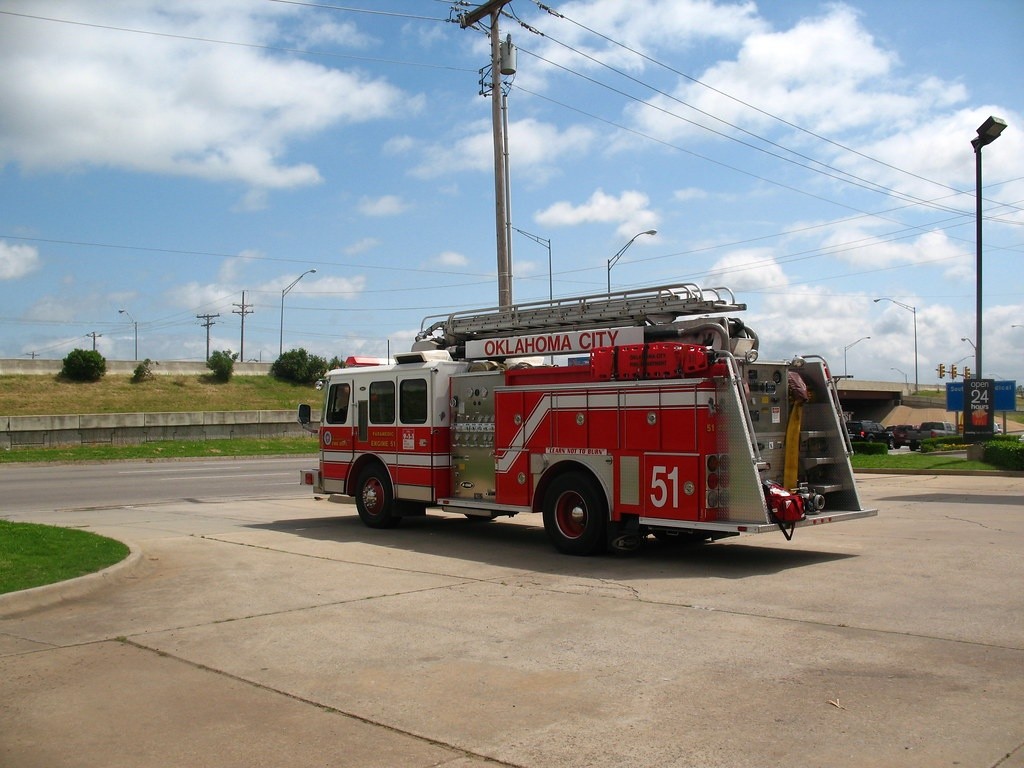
768,481,808,540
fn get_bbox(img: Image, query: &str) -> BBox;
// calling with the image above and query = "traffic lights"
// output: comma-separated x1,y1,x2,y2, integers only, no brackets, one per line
952,364,958,380
964,366,971,380
940,364,946,378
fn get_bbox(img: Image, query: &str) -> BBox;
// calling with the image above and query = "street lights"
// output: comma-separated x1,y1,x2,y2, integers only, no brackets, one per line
845,336,870,381
606,228,658,298
117,308,139,361
889,367,908,385
968,115,1007,386
990,373,1007,434
279,268,317,356
873,297,921,394
961,336,979,381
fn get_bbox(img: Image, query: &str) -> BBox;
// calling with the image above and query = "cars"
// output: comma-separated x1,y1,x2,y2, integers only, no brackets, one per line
845,417,1003,452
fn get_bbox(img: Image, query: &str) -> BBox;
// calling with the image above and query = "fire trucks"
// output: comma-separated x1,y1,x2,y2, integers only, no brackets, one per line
297,280,879,558
